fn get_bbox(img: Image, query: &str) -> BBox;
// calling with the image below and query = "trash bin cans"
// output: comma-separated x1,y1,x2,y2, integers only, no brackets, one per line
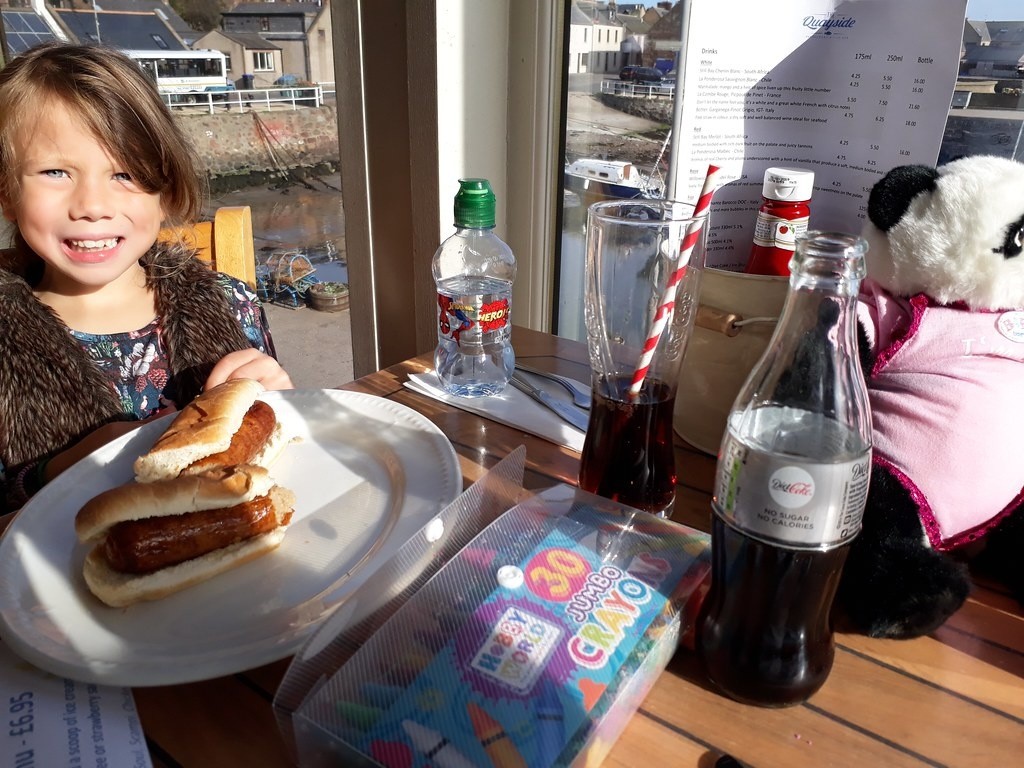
242,74,254,90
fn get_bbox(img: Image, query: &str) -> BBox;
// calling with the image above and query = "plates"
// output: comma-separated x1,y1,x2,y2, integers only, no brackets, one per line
0,388,464,690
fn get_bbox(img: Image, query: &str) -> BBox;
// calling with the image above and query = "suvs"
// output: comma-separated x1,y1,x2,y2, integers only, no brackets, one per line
632,67,663,85
619,65,641,81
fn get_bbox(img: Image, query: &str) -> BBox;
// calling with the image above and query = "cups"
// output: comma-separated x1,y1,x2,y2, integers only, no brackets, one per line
576,198,711,521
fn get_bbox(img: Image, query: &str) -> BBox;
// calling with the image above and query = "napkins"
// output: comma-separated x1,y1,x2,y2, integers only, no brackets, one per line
404,367,595,451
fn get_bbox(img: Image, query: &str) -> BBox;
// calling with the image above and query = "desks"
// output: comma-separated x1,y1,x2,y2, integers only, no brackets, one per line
0,324,1023,767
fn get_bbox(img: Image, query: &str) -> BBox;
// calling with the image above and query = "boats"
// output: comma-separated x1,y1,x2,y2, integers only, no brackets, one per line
565,158,650,201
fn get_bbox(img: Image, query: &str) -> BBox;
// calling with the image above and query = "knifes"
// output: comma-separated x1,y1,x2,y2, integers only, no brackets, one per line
509,372,590,433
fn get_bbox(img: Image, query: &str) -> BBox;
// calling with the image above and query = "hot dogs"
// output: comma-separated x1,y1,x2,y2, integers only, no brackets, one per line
134,377,282,485
74,464,295,608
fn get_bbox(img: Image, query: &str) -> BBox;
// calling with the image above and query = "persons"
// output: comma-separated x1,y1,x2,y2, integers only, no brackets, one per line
1,41,299,514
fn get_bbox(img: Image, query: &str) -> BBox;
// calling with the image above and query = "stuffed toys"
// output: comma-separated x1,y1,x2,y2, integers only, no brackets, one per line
754,151,1024,641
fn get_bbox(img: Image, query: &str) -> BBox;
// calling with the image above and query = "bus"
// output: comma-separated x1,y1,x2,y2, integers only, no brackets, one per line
116,49,236,107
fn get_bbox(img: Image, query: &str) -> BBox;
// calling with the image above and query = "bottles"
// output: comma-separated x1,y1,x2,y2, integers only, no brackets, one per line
749,164,815,276
698,231,875,709
430,177,518,398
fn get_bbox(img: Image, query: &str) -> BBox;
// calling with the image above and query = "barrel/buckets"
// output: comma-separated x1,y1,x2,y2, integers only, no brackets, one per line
675,236,799,456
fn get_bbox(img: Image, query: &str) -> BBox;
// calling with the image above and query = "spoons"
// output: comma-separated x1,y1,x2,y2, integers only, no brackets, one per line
514,358,593,408
